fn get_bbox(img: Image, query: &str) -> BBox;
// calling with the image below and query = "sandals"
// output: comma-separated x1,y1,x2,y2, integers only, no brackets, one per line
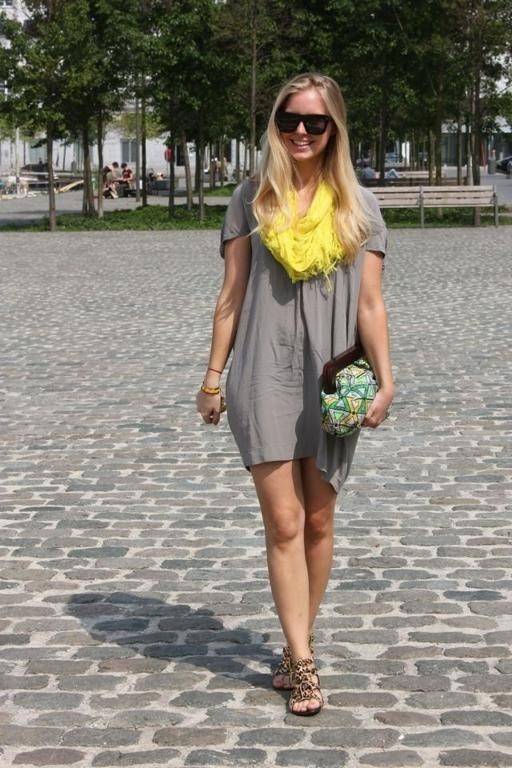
269,630,317,692
285,648,325,720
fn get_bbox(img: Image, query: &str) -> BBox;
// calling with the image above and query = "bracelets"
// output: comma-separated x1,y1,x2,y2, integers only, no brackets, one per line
206,367,223,374
200,383,221,394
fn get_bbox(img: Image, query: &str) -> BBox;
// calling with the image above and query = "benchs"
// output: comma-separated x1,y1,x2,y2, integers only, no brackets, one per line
367,185,498,229
364,171,464,184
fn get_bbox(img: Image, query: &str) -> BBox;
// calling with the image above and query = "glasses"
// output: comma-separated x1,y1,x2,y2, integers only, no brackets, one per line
274,110,332,134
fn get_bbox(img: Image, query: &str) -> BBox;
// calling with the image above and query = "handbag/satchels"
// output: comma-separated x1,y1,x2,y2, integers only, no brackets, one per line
319,347,392,438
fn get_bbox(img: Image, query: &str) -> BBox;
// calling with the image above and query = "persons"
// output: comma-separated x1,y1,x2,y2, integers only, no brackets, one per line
156,174,165,181
92,161,134,199
204,155,229,182
387,165,406,179
146,173,154,185
360,162,377,179
196,72,395,717
